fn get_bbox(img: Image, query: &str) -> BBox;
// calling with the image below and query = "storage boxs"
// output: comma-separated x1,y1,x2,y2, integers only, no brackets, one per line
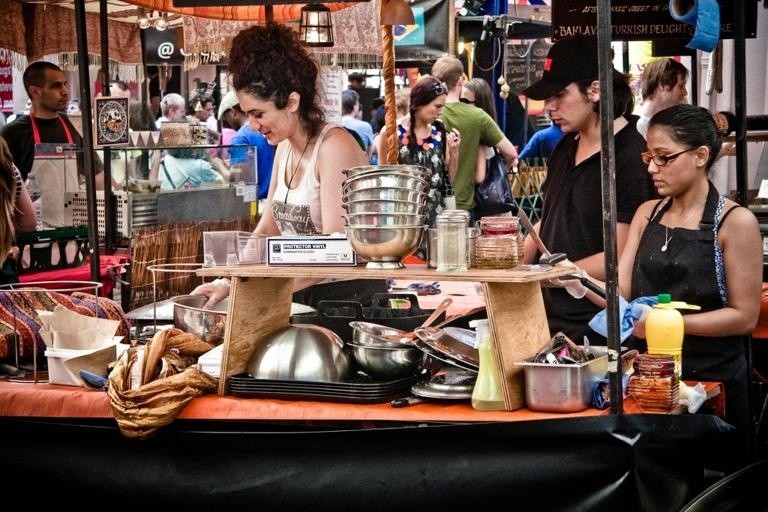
514,338,610,411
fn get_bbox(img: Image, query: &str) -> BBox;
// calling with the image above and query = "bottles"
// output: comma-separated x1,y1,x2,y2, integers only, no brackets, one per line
430,209,477,274
644,294,685,383
626,355,679,413
468,319,507,410
26,171,43,232
478,215,525,269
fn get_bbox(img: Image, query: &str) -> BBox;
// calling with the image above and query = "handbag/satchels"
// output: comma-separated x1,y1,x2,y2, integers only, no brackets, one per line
473,146,518,216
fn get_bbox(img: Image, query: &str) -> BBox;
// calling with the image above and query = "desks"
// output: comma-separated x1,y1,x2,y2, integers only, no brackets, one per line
0,375,730,511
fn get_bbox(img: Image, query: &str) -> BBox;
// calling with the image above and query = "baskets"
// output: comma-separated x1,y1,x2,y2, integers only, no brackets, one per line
13,224,88,275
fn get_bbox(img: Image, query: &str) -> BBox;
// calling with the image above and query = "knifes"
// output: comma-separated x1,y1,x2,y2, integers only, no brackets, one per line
518,207,568,267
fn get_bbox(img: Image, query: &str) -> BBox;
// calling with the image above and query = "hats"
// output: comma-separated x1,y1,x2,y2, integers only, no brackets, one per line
521,34,597,100
217,91,240,121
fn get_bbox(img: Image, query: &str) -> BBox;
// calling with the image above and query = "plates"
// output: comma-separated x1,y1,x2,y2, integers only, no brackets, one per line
411,323,478,400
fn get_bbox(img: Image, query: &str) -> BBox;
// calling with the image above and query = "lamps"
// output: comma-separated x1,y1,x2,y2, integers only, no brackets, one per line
292,0,334,49
133,3,171,32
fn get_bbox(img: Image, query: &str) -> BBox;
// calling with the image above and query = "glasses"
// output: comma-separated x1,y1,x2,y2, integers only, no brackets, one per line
640,147,696,166
425,81,448,94
459,97,475,103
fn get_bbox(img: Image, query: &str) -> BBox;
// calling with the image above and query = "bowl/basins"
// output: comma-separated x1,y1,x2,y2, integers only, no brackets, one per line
169,293,228,345
338,163,430,269
247,320,428,382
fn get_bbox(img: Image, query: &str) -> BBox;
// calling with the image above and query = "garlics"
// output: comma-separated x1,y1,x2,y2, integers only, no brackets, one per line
497,74,510,100
